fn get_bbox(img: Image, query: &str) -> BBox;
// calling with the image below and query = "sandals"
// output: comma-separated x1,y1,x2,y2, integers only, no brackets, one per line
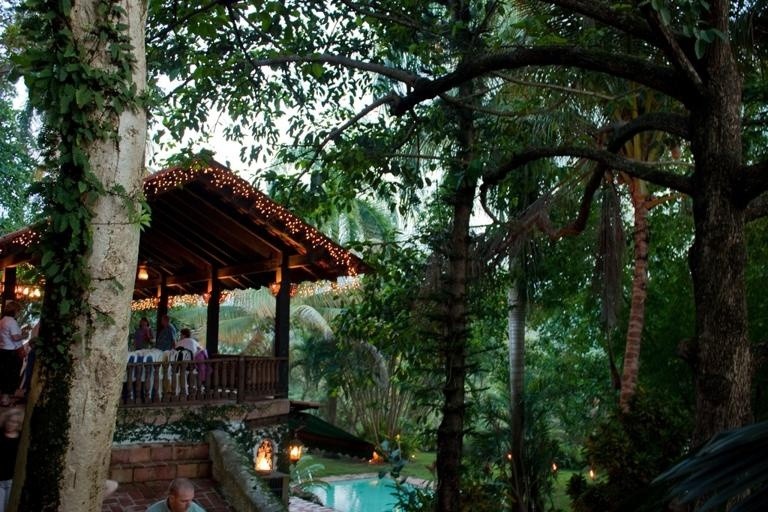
0,399,16,408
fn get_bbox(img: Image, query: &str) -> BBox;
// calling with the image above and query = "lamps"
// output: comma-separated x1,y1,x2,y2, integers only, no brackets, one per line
289,437,305,468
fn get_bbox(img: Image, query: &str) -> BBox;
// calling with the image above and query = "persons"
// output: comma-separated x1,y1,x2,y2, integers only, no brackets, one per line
145,477,208,512
0,409,24,512
134,314,204,361
0,302,40,408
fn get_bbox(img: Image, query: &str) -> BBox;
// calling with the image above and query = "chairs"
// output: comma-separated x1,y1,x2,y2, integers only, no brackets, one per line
126,346,209,398
